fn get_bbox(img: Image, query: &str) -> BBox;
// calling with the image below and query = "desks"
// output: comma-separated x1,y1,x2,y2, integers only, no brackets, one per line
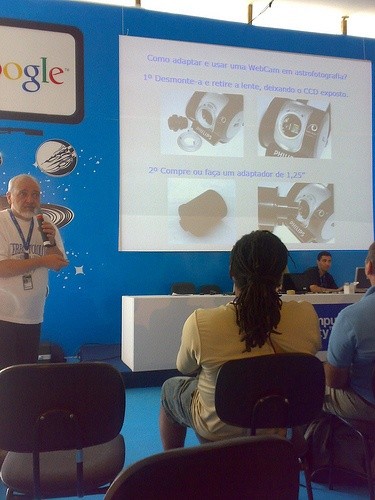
121,294,364,373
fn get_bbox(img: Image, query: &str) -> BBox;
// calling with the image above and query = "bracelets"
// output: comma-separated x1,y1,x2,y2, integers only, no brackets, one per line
47,243,55,247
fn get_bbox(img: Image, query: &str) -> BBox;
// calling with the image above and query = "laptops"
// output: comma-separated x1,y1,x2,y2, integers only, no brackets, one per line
279,273,304,293
354,267,371,293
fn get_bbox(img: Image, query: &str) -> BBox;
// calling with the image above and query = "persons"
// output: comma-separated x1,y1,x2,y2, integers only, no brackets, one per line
323,243,375,423
297,251,344,293
158,229,323,452
0,174,69,369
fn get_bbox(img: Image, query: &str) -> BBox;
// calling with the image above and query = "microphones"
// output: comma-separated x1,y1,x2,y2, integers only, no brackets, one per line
34,206,51,247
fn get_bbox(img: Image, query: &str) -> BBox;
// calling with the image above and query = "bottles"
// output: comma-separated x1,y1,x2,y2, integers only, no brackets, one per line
344,282,350,294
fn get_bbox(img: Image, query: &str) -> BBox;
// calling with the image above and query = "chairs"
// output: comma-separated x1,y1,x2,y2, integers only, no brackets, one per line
215,353,326,500
172,283,222,293
327,414,375,500
103,434,298,500
285,268,308,293
0,362,125,500
